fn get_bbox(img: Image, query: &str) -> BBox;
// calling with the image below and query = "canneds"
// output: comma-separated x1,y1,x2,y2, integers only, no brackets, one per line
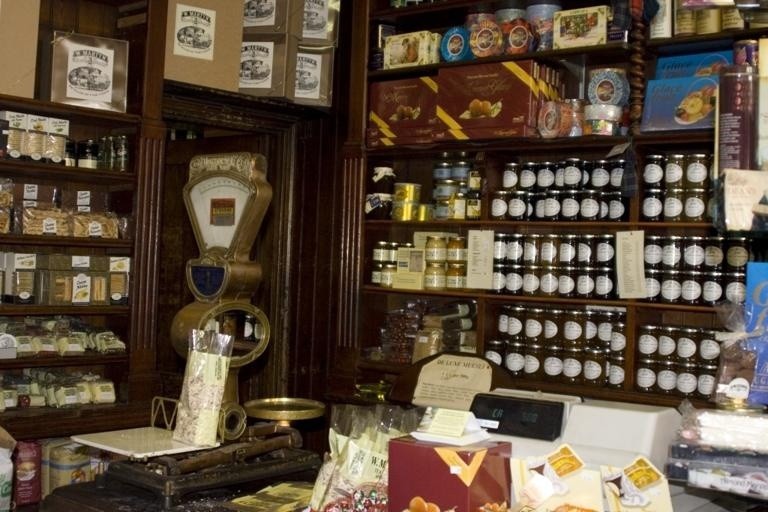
59,140,100,170
480,307,721,401
370,232,753,307
391,151,715,222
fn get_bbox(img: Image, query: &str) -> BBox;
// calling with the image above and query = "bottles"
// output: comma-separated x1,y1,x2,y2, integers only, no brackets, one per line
96,135,129,172
719,65,758,176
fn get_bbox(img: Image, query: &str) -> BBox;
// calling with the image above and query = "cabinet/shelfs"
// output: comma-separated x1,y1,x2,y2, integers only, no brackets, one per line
326,1,768,414
0,0,302,442
